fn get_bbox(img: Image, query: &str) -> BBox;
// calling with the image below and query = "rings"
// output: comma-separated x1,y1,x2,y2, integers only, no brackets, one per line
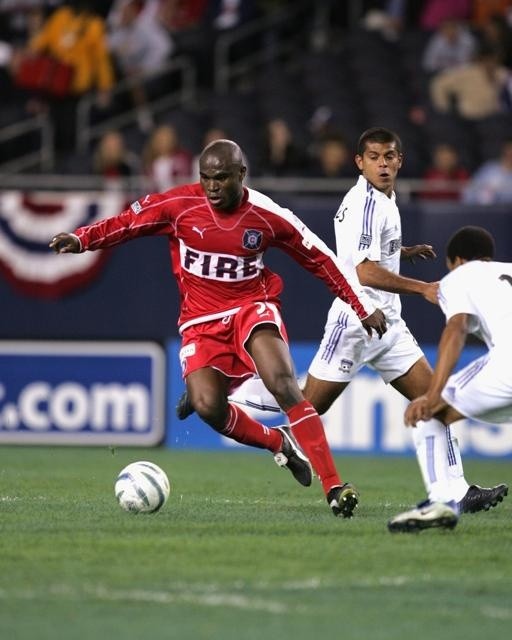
52,236,58,242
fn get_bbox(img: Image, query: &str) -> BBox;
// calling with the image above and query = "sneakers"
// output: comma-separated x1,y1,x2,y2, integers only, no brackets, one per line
387,499,458,533
326,483,360,519
176,391,195,420
458,484,507,514
271,424,314,486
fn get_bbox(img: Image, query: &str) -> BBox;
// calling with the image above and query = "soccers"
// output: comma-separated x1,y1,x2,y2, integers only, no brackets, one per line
115,461,169,514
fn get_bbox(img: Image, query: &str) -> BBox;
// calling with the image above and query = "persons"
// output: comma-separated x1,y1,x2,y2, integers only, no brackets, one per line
177,125,507,517
0,0,511,206
386,214,511,532
46,139,389,523
